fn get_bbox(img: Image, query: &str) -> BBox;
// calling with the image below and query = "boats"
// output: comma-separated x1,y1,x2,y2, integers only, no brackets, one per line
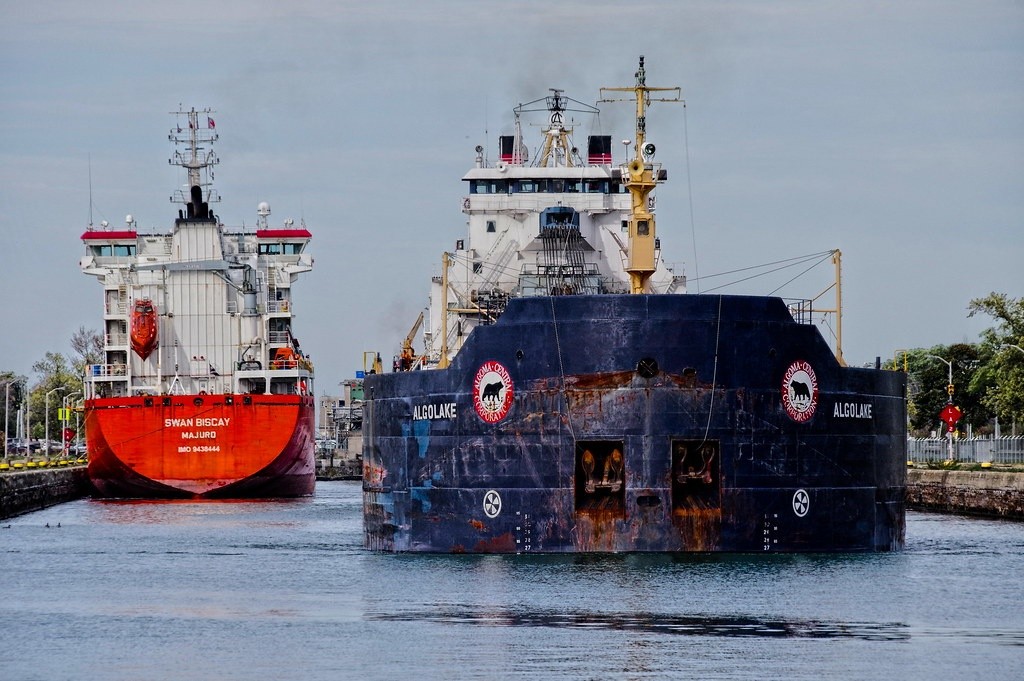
359,56,909,575
66,98,319,500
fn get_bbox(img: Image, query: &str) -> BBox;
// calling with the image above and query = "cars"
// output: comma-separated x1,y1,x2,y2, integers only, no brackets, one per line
4,434,90,457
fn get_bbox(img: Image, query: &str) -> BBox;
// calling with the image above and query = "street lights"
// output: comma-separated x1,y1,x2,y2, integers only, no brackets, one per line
75,397,86,456
924,351,959,463
43,387,66,460
5,378,21,457
61,393,82,456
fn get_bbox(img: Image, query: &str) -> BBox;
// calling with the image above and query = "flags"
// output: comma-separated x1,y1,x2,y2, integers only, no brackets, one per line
208,118,215,129
